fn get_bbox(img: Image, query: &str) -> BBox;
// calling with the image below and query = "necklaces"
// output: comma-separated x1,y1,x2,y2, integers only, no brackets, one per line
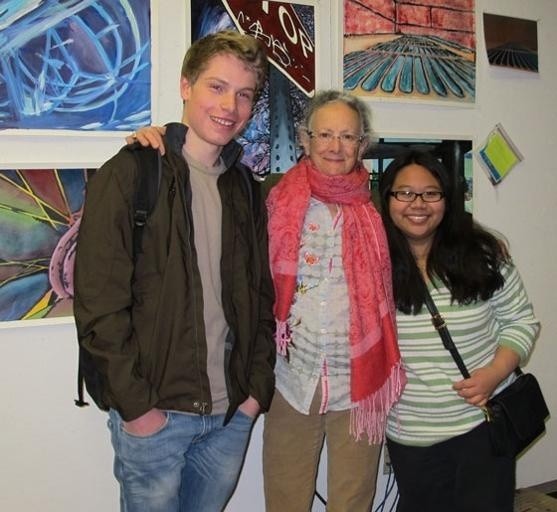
405,230,437,276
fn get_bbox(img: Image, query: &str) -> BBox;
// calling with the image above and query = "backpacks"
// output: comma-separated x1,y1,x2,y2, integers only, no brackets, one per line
77,342,111,413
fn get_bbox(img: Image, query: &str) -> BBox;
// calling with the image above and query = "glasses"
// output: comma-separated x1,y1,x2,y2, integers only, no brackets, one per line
387,188,449,203
304,128,367,146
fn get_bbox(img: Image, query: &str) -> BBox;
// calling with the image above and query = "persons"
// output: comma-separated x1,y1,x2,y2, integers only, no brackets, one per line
374,149,541,512
73,27,275,512
126,87,407,512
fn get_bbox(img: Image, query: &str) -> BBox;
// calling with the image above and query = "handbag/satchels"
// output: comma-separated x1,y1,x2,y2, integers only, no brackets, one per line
482,371,551,459
